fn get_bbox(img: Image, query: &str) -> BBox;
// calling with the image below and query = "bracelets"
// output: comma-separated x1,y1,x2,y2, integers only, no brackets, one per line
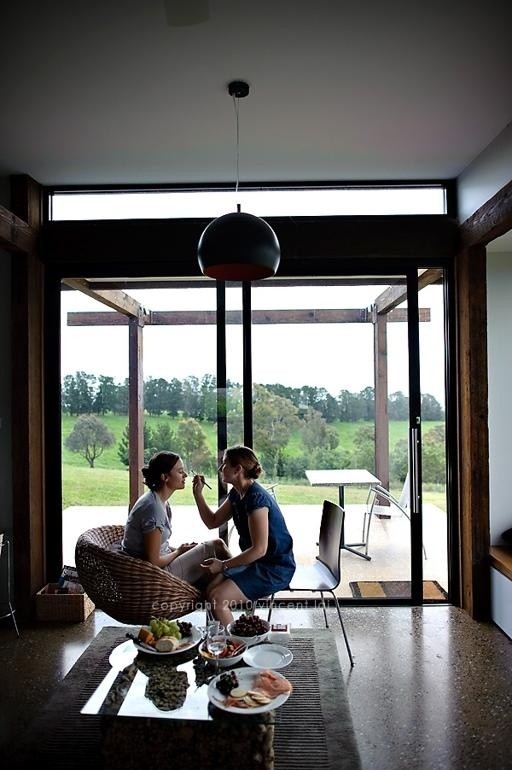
221,559,232,571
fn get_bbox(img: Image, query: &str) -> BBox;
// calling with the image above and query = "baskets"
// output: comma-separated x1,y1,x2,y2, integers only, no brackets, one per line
35,582,96,624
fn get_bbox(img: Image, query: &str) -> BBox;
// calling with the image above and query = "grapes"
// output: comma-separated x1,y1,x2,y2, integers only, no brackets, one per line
151,616,181,640
231,614,268,637
216,671,239,695
178,622,192,638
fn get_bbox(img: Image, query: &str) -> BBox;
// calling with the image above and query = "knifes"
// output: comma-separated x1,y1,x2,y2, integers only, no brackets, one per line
126,632,157,653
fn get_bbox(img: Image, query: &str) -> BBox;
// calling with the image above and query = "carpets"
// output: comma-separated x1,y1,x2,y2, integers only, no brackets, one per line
348,579,450,603
8,622,365,769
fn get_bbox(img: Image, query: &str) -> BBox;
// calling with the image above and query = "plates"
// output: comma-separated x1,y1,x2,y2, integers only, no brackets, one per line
133,619,204,656
242,643,294,670
207,666,291,714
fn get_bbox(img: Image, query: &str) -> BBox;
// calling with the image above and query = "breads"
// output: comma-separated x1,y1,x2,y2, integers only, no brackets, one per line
155,636,179,652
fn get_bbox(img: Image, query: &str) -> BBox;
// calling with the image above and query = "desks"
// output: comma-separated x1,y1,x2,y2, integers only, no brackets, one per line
304,467,383,562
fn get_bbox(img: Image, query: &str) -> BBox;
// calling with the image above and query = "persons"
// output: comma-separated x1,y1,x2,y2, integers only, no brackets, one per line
192,444,297,635
119,450,242,620
133,657,191,712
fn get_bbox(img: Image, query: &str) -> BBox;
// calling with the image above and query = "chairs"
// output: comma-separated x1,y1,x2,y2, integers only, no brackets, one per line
360,470,428,562
263,498,356,670
1,539,20,637
74,522,216,623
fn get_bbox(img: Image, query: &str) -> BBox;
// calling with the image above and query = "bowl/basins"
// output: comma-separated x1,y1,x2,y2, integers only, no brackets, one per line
199,635,248,666
227,620,271,645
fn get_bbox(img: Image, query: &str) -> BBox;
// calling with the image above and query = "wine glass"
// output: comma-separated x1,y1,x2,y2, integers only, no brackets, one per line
207,623,227,674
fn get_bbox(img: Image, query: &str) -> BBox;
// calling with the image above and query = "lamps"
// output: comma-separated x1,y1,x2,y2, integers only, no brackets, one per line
195,79,285,284
